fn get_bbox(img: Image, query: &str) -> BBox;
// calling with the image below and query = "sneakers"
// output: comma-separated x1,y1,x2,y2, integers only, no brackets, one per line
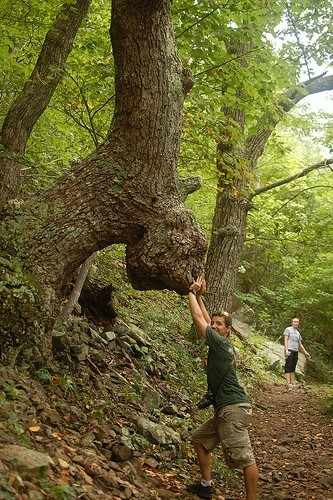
188,481,215,500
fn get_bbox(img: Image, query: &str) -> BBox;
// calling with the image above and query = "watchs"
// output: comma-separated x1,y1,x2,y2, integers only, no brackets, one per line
189,288,196,296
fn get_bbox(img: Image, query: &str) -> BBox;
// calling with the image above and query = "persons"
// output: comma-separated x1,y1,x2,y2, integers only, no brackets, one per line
283,318,310,389
189,276,259,500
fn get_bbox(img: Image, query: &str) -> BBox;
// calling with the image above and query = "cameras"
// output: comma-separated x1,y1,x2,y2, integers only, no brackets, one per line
196,393,213,410
